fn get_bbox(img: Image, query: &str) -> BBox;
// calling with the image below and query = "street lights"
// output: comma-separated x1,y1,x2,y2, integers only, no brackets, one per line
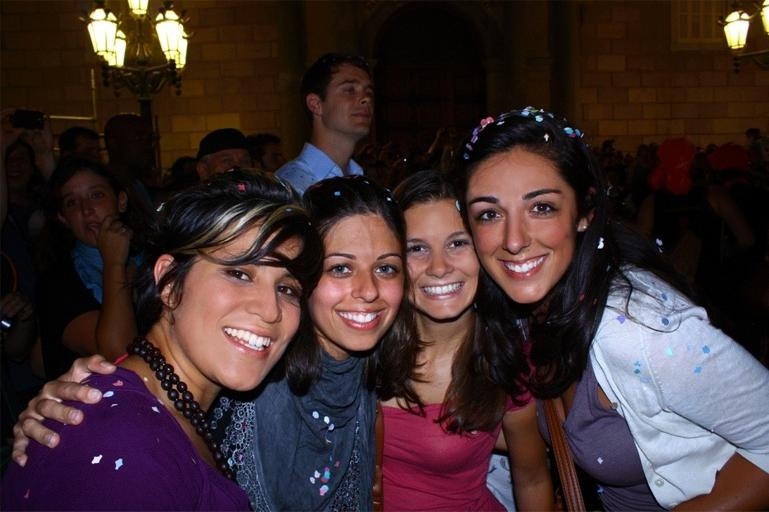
77,0,194,202
718,1,768,68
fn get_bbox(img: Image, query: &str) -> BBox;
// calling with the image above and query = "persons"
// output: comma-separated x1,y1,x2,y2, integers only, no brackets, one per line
585,128,768,370
370,170,556,512
450,102,768,512
14,175,406,511
0,167,324,510
2,54,462,469
